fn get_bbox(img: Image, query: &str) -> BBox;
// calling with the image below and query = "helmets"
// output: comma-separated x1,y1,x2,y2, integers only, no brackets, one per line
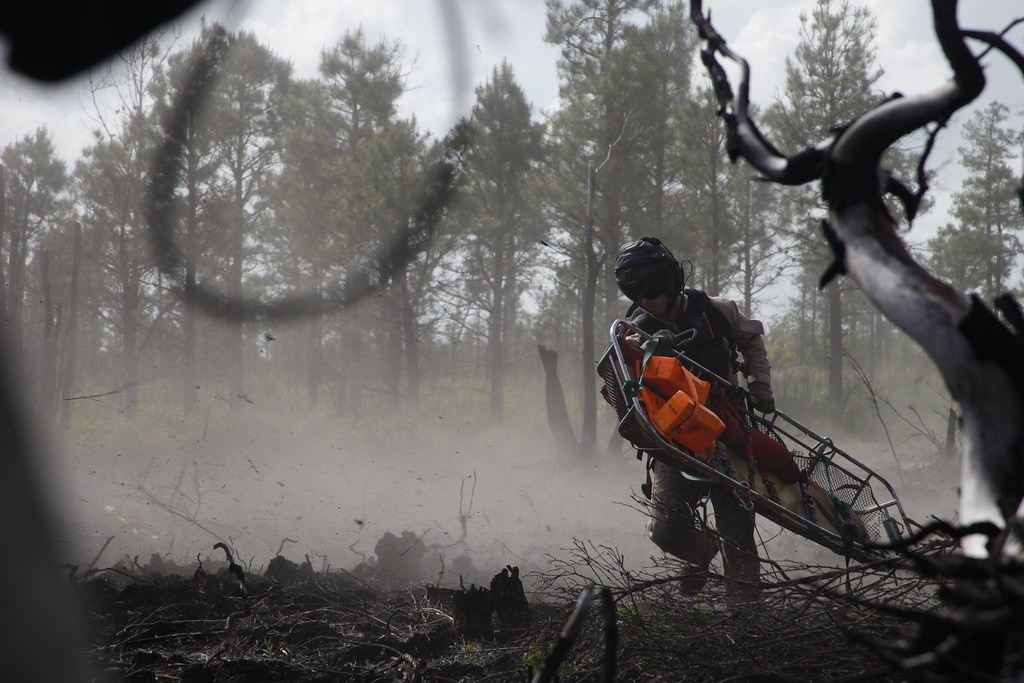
615,236,685,299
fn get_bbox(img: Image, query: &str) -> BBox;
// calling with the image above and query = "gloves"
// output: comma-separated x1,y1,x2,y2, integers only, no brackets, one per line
652,329,678,355
749,381,776,414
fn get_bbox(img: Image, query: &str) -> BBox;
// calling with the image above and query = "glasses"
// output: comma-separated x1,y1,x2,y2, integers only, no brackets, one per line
616,273,668,304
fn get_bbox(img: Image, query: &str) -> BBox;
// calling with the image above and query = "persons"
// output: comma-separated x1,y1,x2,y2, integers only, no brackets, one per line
614,236,776,609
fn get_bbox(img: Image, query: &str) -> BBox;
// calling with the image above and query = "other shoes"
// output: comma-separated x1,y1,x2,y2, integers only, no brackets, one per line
678,535,720,595
